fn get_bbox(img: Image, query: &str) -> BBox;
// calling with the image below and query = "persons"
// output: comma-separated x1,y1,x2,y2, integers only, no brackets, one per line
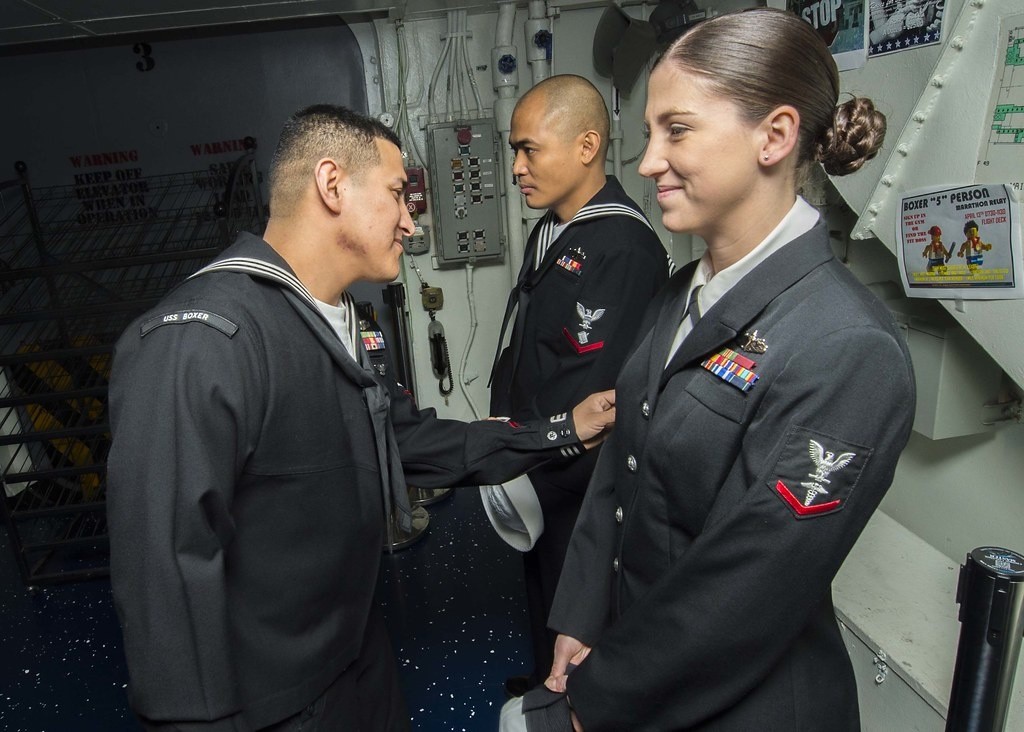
480,74,670,697
499,5,917,732
102,105,617,732
